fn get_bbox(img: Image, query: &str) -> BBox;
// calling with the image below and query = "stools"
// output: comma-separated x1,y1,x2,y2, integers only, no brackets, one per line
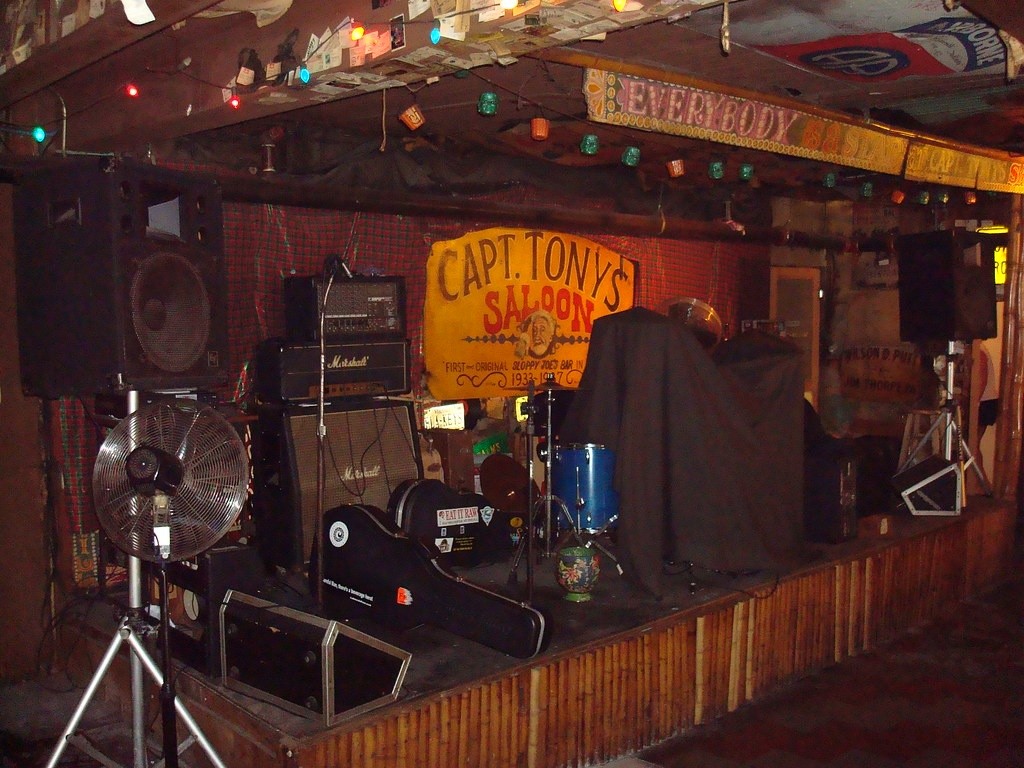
897,409,942,475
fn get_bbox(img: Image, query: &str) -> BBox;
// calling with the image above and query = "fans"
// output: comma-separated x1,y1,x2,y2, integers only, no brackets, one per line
92,398,250,768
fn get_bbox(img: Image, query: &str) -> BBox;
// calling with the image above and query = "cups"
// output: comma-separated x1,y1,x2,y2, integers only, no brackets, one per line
554,547,601,602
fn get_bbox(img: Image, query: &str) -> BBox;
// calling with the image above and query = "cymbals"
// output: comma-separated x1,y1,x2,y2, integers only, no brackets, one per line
658,296,723,350
479,453,542,514
503,380,588,392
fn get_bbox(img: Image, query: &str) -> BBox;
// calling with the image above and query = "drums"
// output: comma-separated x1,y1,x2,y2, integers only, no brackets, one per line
545,437,620,533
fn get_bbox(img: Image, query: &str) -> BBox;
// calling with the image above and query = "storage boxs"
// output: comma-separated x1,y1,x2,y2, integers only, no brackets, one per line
473,430,515,494
420,426,474,491
